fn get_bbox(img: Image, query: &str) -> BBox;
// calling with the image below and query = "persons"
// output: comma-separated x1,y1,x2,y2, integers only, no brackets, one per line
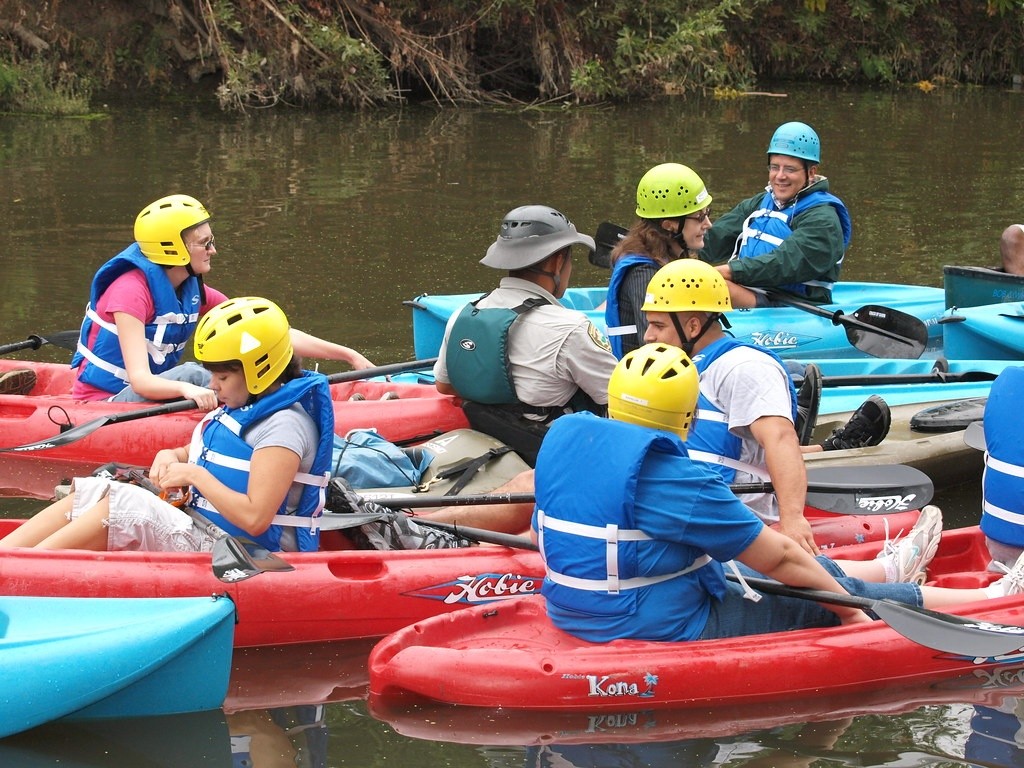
606,162,712,360
0,296,334,553
697,121,851,309
414,258,822,557
529,343,1024,640
433,205,620,471
980,364,1024,563
73,195,390,414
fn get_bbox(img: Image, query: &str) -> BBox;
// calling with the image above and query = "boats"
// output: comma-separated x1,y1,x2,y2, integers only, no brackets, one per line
2,597,236,741
2,265,1022,744
366,578,1023,745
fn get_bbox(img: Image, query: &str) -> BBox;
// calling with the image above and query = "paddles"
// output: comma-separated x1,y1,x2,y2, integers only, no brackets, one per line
407,515,1024,660
794,371,999,389
587,219,930,362
1,356,440,455
370,462,935,518
92,460,299,585
1,328,80,357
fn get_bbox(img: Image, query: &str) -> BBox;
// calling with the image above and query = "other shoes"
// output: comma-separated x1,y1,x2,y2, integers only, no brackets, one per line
347,393,365,401
380,391,400,400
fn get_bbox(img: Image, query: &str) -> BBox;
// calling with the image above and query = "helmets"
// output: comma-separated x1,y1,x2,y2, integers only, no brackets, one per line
635,163,712,218
134,195,210,266
193,297,293,394
766,122,821,166
640,259,734,312
607,343,699,442
476,204,597,269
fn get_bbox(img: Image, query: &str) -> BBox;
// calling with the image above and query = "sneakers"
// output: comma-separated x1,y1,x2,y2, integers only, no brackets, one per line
325,476,415,549
793,363,822,446
987,549,1024,597
386,514,480,550
822,395,891,450
876,505,942,584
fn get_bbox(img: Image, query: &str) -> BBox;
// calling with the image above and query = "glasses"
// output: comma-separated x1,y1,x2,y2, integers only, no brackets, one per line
766,164,805,175
683,207,711,222
187,235,216,251
